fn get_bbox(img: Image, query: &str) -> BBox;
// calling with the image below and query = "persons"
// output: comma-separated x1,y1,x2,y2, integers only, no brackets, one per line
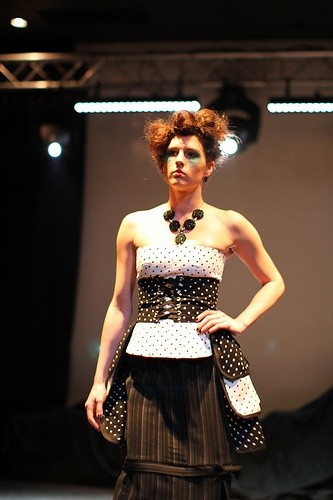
84,109,286,500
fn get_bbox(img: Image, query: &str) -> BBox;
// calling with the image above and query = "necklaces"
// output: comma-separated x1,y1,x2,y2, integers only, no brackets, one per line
163,208,203,245
